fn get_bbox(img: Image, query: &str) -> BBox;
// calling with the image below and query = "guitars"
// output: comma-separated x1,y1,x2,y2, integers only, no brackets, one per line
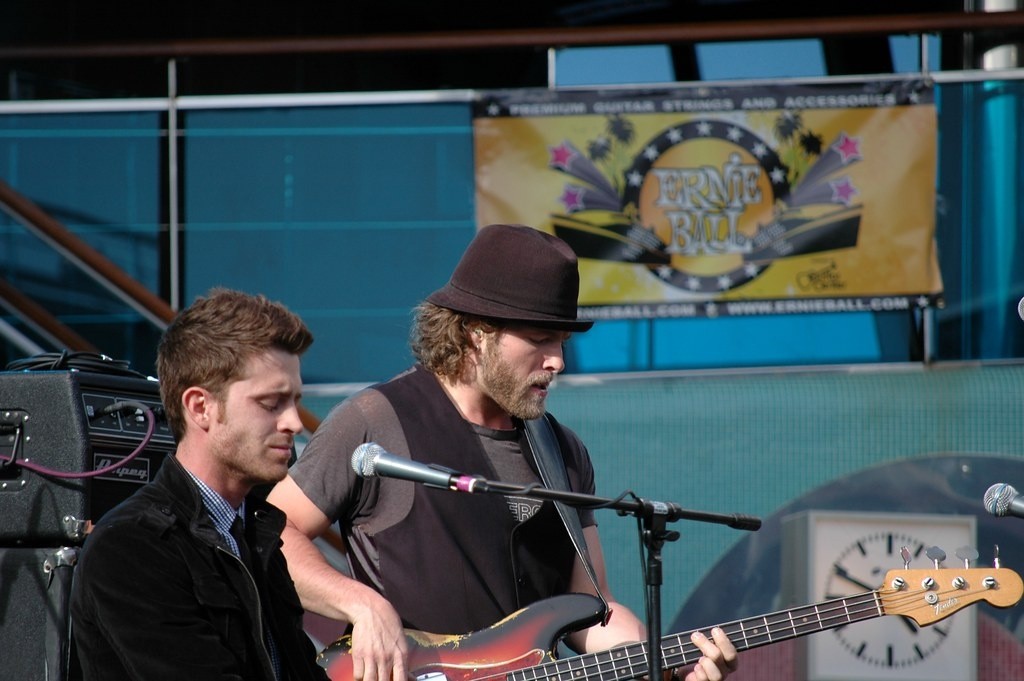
317,541,1024,681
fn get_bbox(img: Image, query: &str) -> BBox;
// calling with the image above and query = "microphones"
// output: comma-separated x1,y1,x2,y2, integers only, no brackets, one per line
983,483,1024,519
351,441,490,494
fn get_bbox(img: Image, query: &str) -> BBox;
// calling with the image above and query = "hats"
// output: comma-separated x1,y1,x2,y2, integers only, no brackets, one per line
425,224,594,332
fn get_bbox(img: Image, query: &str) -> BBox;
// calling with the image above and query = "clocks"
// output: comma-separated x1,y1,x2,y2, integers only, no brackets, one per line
781,509,978,681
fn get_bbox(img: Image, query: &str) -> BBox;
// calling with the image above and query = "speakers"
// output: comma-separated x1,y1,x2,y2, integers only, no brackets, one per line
0,546,85,681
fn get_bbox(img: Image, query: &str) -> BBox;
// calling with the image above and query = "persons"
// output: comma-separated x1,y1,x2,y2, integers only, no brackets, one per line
264,223,737,681
70,289,332,681
